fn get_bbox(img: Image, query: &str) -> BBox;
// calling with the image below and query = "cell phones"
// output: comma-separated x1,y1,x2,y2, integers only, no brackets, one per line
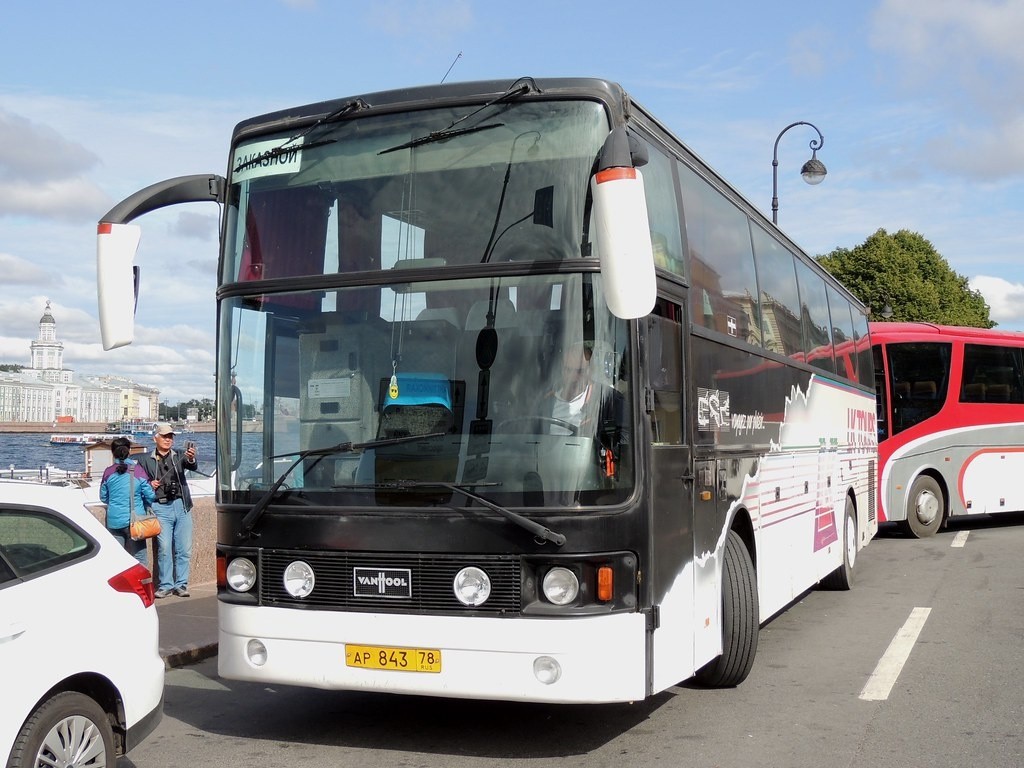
183,440,197,449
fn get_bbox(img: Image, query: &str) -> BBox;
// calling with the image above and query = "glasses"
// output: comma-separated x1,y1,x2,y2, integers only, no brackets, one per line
563,368,585,374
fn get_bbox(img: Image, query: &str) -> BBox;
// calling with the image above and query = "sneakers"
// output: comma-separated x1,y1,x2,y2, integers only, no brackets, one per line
154,590,173,597
174,587,190,597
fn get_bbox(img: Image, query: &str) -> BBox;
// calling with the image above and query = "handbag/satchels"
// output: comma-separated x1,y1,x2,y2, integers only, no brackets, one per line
130,515,163,540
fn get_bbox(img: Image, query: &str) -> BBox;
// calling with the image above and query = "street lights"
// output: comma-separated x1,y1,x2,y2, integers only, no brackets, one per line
771,121,826,226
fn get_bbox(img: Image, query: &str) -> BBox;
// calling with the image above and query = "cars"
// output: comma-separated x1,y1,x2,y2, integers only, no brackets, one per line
1,482,165,768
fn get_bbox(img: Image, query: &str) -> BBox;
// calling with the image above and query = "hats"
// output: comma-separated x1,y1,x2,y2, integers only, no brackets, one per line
157,425,176,436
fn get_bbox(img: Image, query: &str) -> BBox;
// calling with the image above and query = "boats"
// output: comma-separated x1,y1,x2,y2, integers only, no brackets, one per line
119,418,183,437
49,434,134,447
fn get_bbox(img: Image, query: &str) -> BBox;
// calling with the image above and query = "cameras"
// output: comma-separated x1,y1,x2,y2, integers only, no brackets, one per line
164,481,179,500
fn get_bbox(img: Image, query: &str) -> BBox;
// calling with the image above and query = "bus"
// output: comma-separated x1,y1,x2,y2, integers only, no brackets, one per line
867,321,1024,538
97,78,880,707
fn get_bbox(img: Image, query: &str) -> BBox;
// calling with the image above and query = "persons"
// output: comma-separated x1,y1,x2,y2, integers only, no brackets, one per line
530,344,630,462
99,426,197,598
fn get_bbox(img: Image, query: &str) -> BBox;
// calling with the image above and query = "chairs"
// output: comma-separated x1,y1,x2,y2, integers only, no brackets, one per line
457,298,522,377
985,383,1011,402
517,309,555,356
964,384,986,402
356,373,459,484
895,382,911,401
415,307,463,340
903,382,937,421
